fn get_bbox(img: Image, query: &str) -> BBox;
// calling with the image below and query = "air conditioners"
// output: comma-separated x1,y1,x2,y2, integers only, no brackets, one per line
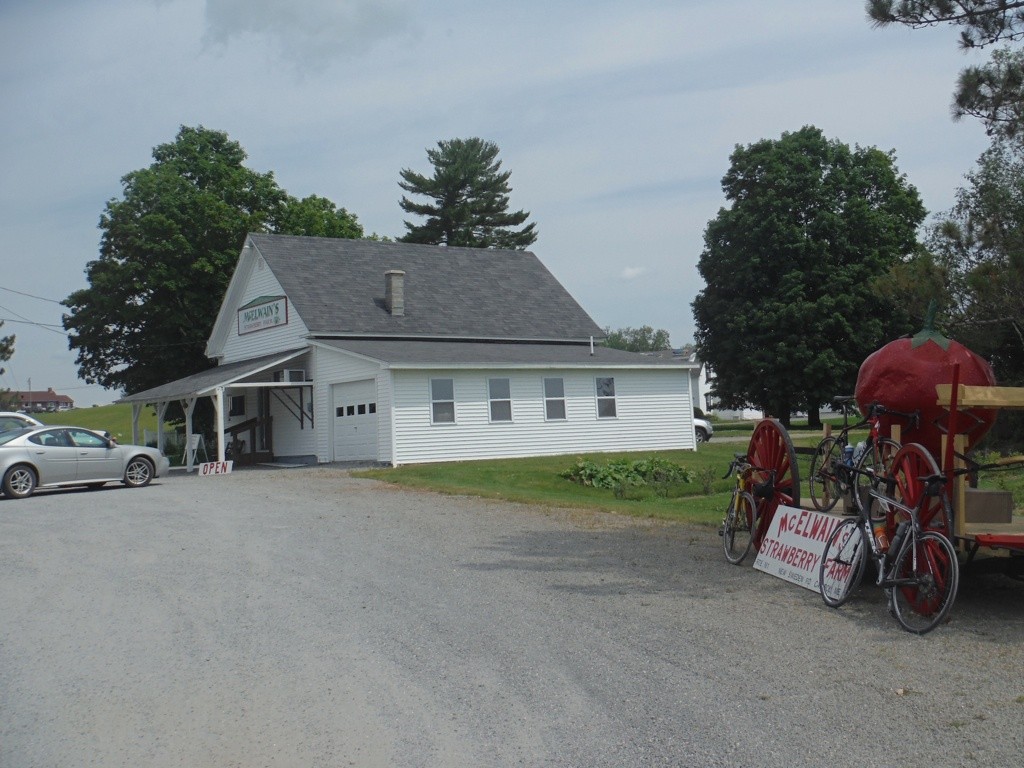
279,367,306,384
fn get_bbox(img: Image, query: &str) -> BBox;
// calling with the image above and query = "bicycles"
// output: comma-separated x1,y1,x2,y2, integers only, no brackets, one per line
819,455,959,635
721,453,765,566
809,395,920,523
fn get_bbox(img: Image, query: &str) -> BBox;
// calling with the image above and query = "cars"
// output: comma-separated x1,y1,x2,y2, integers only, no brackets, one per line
56,406,71,412
0,424,169,499
16,407,27,413
694,417,714,444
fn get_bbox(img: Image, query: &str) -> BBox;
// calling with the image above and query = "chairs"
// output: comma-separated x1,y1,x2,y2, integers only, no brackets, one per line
31,437,43,445
45,436,58,446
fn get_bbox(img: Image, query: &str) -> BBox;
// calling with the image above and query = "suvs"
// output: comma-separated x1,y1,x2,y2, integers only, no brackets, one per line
0,412,109,446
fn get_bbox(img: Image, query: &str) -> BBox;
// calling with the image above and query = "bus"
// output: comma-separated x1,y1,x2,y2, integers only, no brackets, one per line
21,400,59,413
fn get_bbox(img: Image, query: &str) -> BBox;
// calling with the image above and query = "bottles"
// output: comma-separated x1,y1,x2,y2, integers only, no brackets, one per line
874,525,890,553
844,442,854,467
852,441,865,464
